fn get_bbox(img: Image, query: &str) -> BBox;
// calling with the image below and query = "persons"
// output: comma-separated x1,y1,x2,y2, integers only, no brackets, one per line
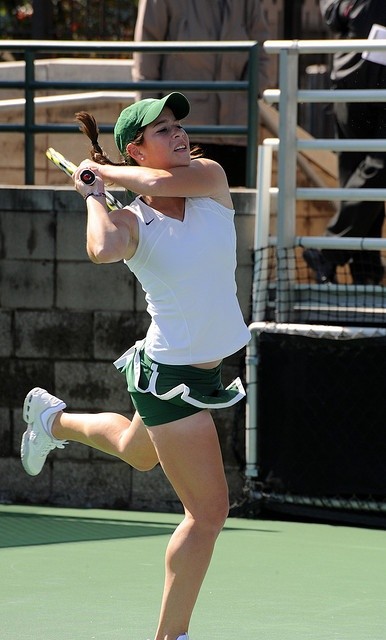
20,90,253,640
132,2,270,188
301,2,386,286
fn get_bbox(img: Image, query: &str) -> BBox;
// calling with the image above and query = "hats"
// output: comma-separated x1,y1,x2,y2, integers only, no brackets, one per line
114,92,190,157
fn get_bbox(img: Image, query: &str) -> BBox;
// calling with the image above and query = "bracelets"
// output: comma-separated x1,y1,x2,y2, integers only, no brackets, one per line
82,191,107,200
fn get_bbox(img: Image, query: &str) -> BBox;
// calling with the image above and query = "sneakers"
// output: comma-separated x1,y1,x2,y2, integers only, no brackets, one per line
20,387,70,476
